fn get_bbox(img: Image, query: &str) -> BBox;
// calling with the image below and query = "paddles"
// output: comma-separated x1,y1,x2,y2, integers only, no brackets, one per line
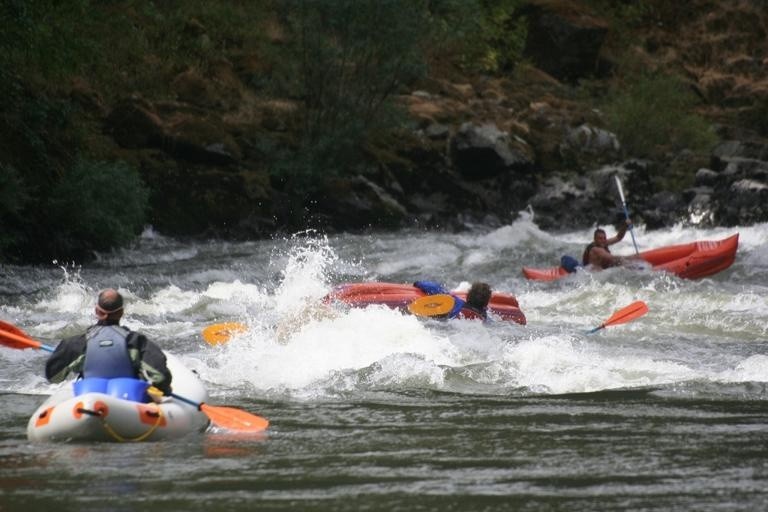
4,322,269,435
614,174,643,272
203,294,455,348
584,300,650,337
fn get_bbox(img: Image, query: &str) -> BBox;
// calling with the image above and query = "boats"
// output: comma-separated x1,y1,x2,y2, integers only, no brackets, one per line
522,233,738,282
321,283,526,325
26,350,209,443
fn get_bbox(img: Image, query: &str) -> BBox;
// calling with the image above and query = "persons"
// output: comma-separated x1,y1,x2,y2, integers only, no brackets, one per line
45,288,172,397
583,218,631,272
414,280,492,321
